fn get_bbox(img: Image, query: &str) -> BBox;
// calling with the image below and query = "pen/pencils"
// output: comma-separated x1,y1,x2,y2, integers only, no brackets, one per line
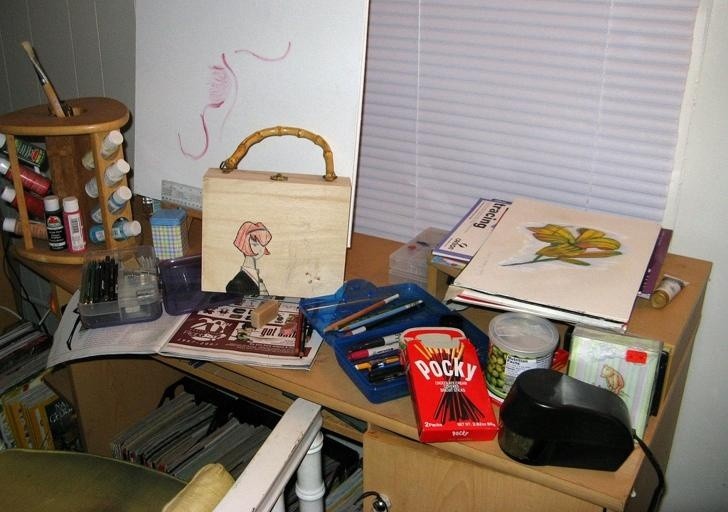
295,294,424,383
82,256,121,322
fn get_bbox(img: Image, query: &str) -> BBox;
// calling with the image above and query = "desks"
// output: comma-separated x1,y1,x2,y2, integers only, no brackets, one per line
7,197,712,511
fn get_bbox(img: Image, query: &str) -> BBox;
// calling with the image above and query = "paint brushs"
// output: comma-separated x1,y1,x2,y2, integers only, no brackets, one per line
22,41,81,118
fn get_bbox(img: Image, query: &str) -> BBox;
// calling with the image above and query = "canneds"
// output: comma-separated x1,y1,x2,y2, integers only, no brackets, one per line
484,312,560,400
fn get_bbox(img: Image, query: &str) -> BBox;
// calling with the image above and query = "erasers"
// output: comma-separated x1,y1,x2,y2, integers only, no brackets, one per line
250,300,279,328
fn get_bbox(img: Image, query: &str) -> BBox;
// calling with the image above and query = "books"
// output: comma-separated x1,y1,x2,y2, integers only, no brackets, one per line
108,392,272,485
2,321,85,455
285,444,364,512
45,290,326,372
432,196,675,332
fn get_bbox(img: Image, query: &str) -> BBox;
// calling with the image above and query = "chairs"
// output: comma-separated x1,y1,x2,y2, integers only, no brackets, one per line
0,398,329,512
